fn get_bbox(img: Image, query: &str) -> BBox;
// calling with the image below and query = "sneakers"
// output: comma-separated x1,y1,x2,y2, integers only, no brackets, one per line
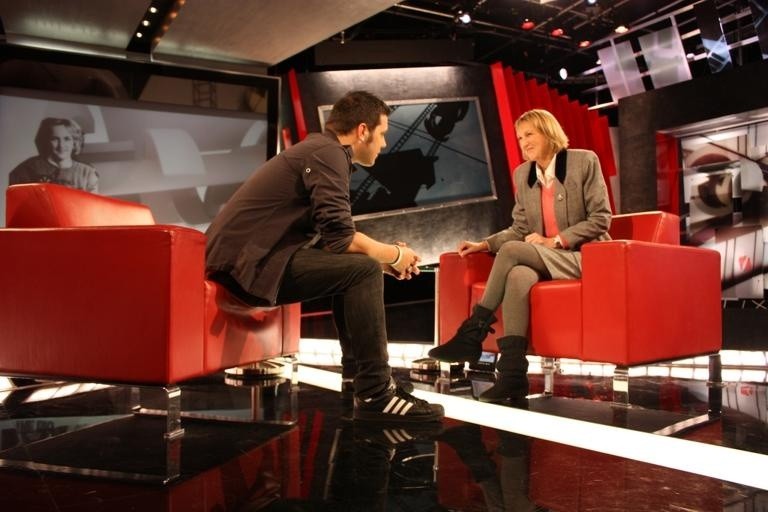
354,423,444,461
341,380,445,423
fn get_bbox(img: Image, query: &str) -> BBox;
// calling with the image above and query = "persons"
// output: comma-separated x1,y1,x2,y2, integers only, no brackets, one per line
8,115,103,197
435,394,552,511
202,89,445,424
424,107,614,402
320,415,444,510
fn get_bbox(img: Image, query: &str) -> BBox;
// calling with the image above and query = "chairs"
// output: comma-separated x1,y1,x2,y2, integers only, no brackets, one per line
436,212,723,436
0,183,308,486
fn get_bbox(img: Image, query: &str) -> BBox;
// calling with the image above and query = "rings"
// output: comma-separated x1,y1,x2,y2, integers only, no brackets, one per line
407,265,414,274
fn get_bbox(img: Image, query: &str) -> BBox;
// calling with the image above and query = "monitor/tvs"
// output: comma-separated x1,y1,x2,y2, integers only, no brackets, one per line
0,86,268,234
0,46,281,234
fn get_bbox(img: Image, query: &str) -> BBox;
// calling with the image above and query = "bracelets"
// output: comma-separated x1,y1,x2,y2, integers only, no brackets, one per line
388,244,403,267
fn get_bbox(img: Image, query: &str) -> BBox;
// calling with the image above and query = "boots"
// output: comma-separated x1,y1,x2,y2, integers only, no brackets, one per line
428,302,499,361
433,422,532,464
479,336,532,401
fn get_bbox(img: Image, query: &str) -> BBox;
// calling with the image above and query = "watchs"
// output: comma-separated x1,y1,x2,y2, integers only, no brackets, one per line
551,236,560,249
